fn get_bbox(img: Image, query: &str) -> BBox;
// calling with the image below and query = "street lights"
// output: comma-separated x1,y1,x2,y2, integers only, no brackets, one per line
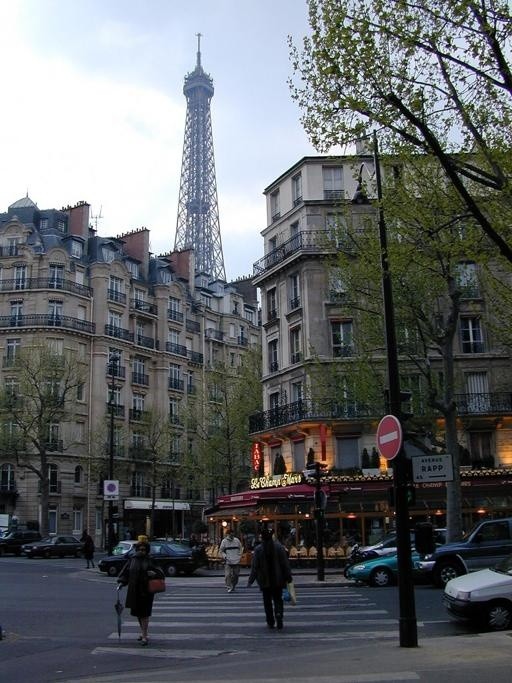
107,348,121,556
350,126,424,649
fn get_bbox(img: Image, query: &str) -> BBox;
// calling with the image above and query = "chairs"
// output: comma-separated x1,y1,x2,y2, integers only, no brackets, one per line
203,542,353,569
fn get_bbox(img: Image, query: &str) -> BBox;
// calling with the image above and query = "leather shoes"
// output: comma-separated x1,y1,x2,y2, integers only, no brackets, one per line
136,631,144,643
140,638,147,647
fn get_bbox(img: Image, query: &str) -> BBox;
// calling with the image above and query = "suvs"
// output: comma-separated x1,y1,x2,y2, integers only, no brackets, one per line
0,529,42,557
414,517,512,585
344,525,465,563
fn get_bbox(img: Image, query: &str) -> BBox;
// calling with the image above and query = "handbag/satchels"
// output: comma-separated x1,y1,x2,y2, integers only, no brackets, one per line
283,582,298,605
146,578,166,593
80,543,85,548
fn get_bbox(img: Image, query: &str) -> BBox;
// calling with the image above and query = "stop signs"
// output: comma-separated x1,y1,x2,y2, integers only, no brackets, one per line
373,412,406,460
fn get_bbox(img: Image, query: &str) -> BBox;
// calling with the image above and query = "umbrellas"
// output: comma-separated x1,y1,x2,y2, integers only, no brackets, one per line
114,590,125,644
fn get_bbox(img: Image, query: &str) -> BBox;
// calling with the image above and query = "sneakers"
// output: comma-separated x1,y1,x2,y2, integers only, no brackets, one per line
227,587,232,594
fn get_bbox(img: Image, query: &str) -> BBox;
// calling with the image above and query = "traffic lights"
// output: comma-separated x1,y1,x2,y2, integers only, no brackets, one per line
312,508,325,519
308,460,318,487
399,388,419,442
319,460,331,486
387,484,417,506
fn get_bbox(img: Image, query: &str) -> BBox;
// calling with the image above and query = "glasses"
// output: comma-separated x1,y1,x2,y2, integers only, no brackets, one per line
135,548,146,551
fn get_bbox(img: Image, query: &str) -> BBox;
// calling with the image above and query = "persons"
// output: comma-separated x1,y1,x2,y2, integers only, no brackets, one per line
218,528,242,593
245,529,294,629
175,532,186,540
79,528,96,568
124,534,148,559
116,540,166,646
188,532,199,549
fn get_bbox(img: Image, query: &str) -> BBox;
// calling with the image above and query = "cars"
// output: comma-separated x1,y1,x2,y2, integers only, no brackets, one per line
96,540,208,575
342,543,447,588
157,537,188,543
20,532,86,559
113,538,139,555
440,553,512,633
